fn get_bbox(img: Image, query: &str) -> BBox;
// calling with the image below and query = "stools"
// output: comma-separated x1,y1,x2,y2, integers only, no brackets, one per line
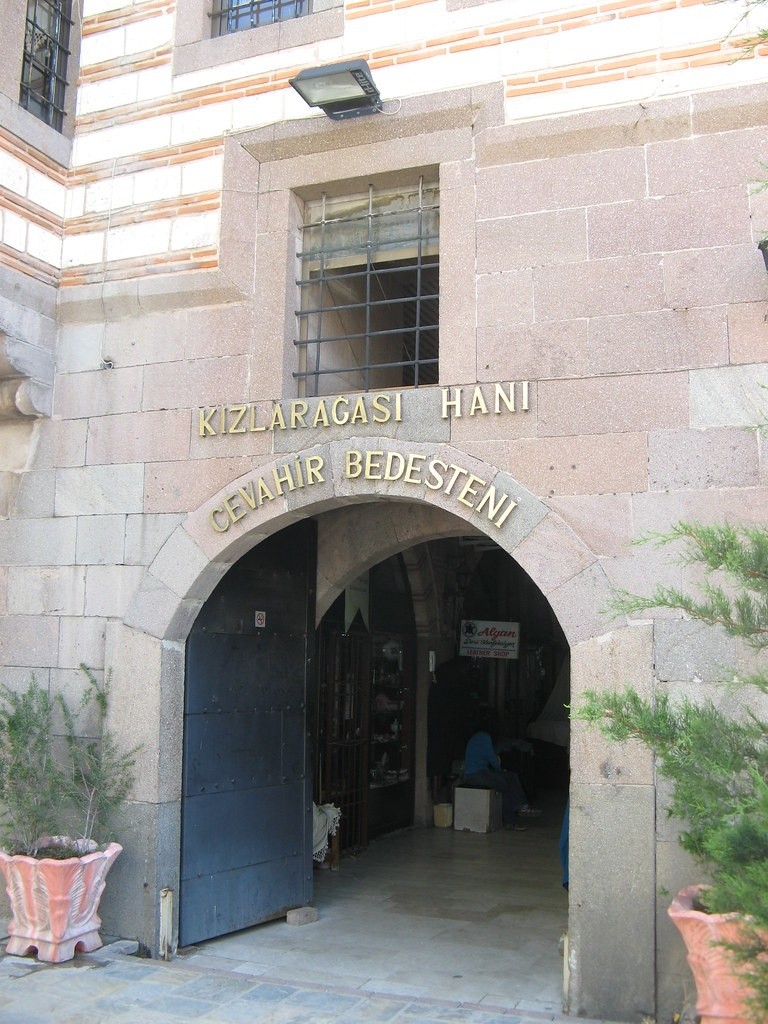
454,786,503,834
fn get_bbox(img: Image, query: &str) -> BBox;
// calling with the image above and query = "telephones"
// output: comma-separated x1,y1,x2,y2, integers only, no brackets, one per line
428,650,436,673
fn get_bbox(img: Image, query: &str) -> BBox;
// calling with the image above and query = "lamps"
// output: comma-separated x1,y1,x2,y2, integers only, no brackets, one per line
446,560,474,600
289,59,383,120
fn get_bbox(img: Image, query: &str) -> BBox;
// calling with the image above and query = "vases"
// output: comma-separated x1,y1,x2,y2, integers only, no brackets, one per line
667,885,768,1024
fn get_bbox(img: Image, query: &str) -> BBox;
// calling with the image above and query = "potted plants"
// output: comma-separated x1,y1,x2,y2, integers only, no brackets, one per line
0,662,146,961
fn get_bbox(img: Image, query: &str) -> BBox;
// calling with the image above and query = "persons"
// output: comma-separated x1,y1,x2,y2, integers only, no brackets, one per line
461,715,544,833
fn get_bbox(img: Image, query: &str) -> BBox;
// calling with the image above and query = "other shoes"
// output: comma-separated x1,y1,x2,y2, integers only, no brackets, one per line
521,808,542,817
509,825,528,831
